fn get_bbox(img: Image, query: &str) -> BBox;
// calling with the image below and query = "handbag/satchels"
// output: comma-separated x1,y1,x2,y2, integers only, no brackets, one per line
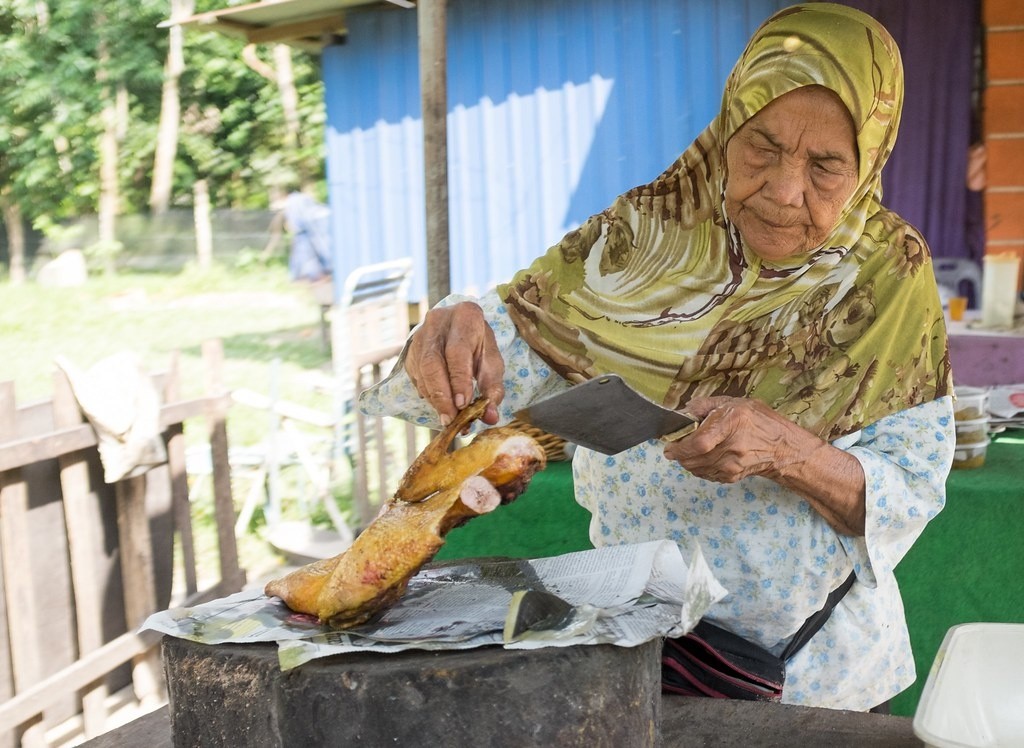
661,620,787,704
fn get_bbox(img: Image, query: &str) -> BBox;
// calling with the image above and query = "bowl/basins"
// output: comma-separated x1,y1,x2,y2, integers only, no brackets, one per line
912,622,1024,748
952,386,992,468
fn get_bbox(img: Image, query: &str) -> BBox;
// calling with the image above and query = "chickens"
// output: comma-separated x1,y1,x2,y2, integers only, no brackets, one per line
262,396,547,631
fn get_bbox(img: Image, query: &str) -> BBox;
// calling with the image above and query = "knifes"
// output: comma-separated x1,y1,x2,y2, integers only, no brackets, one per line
512,374,703,456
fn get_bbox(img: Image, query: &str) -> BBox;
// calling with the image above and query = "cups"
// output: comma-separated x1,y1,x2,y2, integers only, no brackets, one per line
949,297,967,321
981,251,1021,328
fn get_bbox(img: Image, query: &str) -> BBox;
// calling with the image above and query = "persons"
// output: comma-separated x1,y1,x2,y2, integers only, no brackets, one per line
258,182,330,261
356,0,957,718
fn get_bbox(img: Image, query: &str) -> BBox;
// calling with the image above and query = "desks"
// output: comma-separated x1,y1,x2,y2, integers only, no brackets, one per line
70,691,924,748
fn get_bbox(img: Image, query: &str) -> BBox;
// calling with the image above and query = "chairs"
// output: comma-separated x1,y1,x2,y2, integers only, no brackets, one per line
231,256,415,561
